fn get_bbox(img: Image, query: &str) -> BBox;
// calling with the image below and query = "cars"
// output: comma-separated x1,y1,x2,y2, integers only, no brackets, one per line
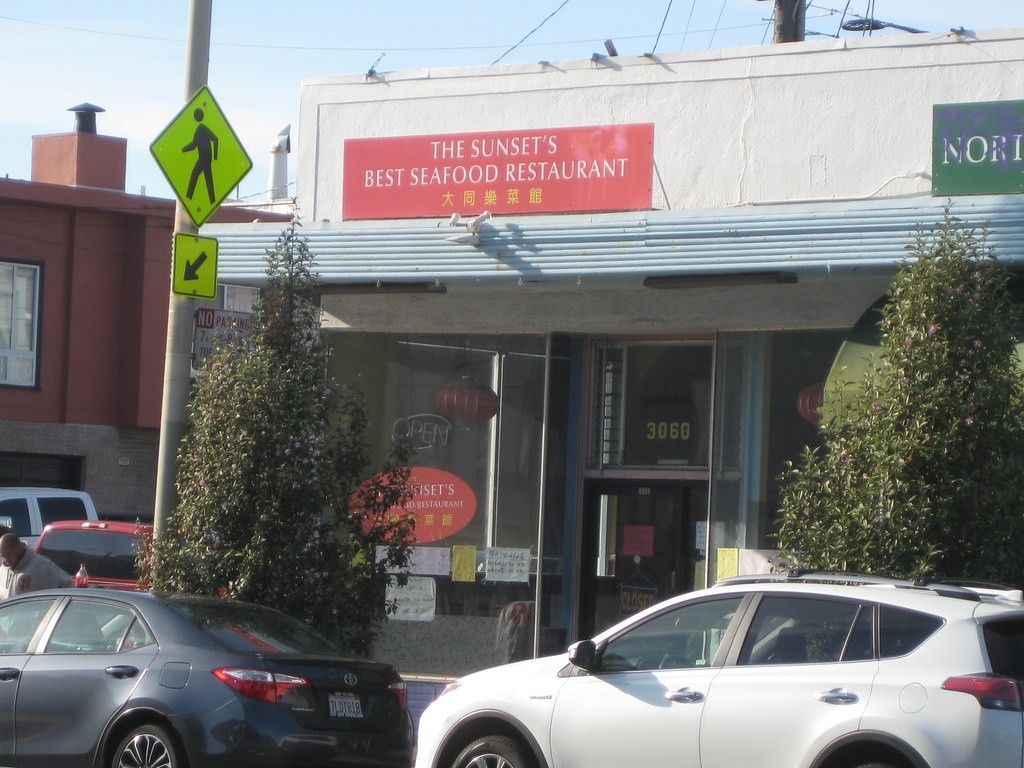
0,590,412,767
411,574,1023,767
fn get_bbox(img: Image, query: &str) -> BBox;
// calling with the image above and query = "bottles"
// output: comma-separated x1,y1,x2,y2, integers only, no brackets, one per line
72,563,88,589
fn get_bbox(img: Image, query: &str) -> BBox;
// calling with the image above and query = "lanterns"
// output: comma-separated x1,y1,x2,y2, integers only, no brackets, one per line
431,374,499,432
796,381,825,427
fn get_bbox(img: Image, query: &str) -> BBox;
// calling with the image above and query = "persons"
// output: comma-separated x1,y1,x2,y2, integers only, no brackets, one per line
0,532,75,600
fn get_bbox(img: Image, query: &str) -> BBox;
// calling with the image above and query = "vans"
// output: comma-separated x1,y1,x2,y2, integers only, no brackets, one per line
30,517,224,597
0,485,99,601
